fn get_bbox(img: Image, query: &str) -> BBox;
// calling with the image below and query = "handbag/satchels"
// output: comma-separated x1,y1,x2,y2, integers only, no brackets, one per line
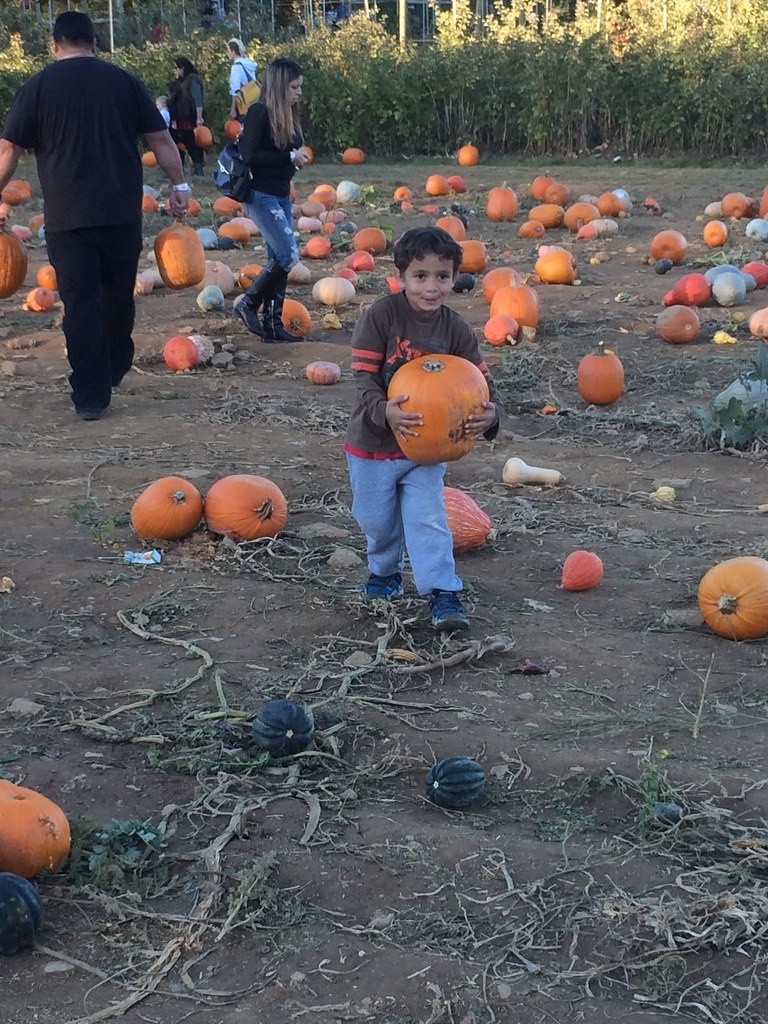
212,103,268,203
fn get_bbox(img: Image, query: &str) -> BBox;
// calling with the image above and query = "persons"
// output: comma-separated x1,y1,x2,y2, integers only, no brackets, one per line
150,18,161,44
201,9,238,29
233,58,311,343
167,58,204,177
226,41,257,125
343,225,503,630
0,11,189,419
328,0,349,21
157,95,170,128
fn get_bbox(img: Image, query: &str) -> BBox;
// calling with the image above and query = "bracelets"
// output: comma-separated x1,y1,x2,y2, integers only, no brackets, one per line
290,152,295,161
172,183,188,191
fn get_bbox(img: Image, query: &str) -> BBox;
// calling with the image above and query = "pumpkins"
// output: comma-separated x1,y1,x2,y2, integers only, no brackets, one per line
424,756,486,808
698,556,768,640
0,121,768,556
0,779,71,879
0,872,46,954
560,550,603,591
252,698,313,756
653,801,683,825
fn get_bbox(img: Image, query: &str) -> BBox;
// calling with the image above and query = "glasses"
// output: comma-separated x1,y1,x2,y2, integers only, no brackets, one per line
174,66,180,69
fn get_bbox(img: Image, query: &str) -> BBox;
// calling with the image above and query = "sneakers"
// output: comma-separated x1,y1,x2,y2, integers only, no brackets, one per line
358,569,405,603
429,586,468,630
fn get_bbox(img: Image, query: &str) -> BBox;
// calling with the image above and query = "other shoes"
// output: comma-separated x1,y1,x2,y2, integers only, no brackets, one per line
75,406,101,421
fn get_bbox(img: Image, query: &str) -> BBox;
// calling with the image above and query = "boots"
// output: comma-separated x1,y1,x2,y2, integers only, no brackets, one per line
232,256,282,338
261,268,306,343
